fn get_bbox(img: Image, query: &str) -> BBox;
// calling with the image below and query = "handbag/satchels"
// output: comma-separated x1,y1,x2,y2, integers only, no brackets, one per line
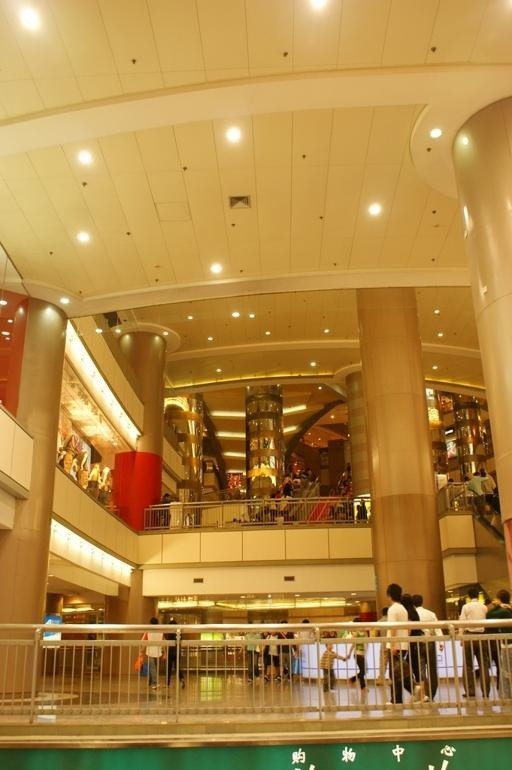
388,652,413,682
134,656,150,677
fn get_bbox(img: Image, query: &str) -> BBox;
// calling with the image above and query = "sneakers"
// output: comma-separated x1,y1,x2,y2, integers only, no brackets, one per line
247,669,289,682
386,685,434,704
151,678,185,689
323,678,369,695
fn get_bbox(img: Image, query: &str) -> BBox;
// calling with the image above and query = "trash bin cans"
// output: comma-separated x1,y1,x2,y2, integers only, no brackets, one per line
169,502,183,530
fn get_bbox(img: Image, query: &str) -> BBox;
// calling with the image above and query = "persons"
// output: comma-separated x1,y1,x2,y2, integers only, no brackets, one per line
231,462,367,523
448,468,498,520
237,584,512,704
57,433,110,502
141,616,166,690
164,620,186,687
161,493,175,527
